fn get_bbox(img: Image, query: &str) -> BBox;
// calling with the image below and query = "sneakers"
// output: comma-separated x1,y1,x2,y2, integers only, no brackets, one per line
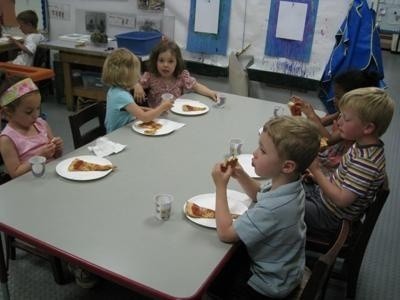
69,262,98,289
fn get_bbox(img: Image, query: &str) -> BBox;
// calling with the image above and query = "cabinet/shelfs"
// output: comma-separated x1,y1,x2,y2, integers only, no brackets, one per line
40,32,164,105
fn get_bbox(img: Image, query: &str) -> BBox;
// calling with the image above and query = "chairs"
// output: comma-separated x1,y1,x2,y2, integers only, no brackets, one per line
69,100,106,150
297,171,390,299
301,218,349,297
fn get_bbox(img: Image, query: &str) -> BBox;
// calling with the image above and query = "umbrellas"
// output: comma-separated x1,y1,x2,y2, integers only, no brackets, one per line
317,0,386,115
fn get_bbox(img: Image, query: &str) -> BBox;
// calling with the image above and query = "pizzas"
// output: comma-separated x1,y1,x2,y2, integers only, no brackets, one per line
137,121,161,134
68,159,116,171
187,201,241,218
288,96,305,115
183,105,206,111
221,157,238,178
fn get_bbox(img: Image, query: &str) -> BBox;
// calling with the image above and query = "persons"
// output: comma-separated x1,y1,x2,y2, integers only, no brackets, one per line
0,71,97,288
132,41,219,109
6,9,46,68
212,114,321,300
101,47,174,135
290,69,378,145
290,86,395,234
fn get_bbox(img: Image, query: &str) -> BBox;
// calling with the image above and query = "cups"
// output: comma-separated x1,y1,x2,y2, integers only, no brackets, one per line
229,138,243,156
154,193,173,220
162,93,174,101
29,156,47,176
219,93,228,107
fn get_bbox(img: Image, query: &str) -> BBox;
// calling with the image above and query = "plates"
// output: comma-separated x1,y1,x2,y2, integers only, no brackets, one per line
133,118,175,135
228,153,268,180
54,155,114,182
1,36,22,42
183,193,249,229
170,99,210,115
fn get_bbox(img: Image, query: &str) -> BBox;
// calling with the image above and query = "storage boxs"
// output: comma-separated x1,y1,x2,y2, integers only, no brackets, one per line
115,31,162,56
